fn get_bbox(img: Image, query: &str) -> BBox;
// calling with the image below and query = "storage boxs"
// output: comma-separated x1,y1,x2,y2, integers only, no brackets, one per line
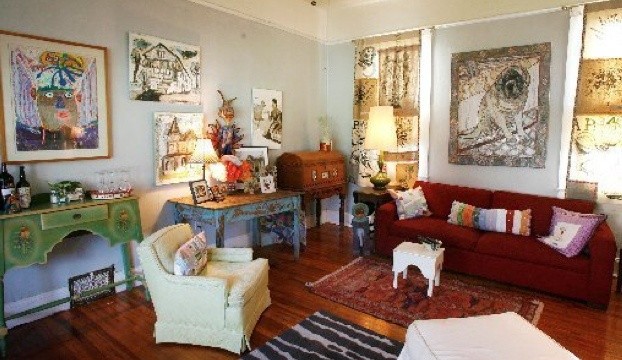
276,149,349,193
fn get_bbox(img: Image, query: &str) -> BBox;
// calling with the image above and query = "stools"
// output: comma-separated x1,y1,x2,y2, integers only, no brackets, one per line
392,241,445,298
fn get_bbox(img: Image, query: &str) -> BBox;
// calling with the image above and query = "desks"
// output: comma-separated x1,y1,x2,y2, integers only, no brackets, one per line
169,185,306,264
277,181,348,245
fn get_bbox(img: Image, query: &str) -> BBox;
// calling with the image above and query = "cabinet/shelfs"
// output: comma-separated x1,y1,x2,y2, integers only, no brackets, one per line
1,193,151,360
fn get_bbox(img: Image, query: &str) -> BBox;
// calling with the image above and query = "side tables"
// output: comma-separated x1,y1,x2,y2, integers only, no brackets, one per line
353,184,407,251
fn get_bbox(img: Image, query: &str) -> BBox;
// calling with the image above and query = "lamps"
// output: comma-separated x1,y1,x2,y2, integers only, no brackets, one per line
362,104,398,190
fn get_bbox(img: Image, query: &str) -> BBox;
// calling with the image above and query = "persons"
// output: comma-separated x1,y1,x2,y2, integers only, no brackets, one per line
262,98,282,144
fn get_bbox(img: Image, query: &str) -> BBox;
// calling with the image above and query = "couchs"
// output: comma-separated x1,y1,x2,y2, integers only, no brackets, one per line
395,312,581,360
371,179,618,311
136,221,275,356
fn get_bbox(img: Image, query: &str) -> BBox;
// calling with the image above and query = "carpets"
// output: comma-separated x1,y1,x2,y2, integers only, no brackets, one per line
304,252,546,352
241,310,407,359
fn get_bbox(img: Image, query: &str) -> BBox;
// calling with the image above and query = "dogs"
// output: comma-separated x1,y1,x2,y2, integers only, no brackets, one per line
458,64,533,144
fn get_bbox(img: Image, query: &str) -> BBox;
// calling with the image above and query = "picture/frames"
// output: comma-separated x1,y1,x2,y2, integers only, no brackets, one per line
188,177,212,205
152,110,208,186
0,30,114,166
248,87,284,151
231,145,271,195
127,30,202,107
446,42,551,170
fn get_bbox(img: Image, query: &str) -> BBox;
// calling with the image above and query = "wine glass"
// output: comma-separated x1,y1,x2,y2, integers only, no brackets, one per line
96,169,131,194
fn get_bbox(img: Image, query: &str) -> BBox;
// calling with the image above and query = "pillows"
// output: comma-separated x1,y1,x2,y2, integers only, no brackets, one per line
447,198,533,238
173,231,208,278
386,185,432,220
535,205,607,258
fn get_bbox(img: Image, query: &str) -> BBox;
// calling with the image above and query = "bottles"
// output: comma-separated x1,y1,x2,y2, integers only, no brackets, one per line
16,166,32,209
0,162,16,211
